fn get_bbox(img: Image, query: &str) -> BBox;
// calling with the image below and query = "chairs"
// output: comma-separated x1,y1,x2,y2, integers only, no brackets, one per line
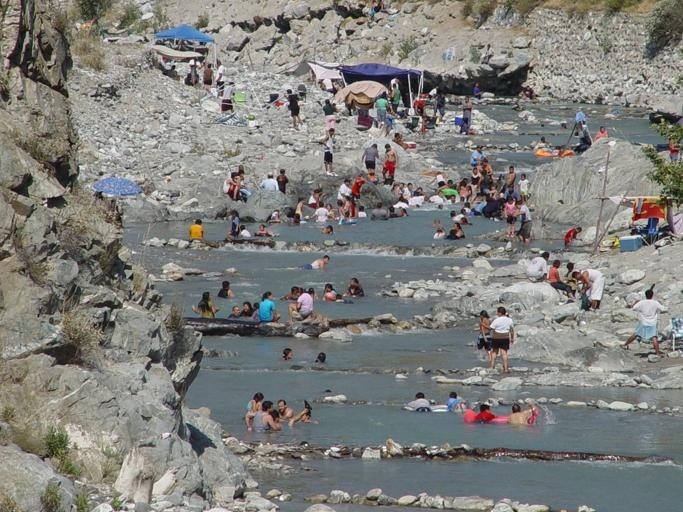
425,116,436,135
634,217,659,248
669,317,682,352
405,116,419,135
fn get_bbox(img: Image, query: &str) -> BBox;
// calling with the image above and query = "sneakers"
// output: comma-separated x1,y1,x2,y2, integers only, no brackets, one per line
506,231,516,240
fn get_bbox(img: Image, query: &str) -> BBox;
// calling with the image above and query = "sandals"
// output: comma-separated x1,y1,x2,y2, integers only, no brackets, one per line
304,399,312,417
622,342,630,352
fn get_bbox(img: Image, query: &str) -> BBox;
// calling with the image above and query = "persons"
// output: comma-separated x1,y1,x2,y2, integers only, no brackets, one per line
476,306,515,373
94,190,124,228
526,250,605,307
621,283,668,356
246,392,319,434
189,124,679,248
367,0,386,20
192,253,364,363
286,76,536,135
402,391,539,426
163,59,235,117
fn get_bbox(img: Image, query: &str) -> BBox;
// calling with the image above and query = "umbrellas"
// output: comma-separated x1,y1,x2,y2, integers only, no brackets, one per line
88,176,144,196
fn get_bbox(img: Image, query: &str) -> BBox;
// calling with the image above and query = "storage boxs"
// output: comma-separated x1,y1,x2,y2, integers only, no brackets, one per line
619,236,638,253
455,115,465,127
633,234,642,248
